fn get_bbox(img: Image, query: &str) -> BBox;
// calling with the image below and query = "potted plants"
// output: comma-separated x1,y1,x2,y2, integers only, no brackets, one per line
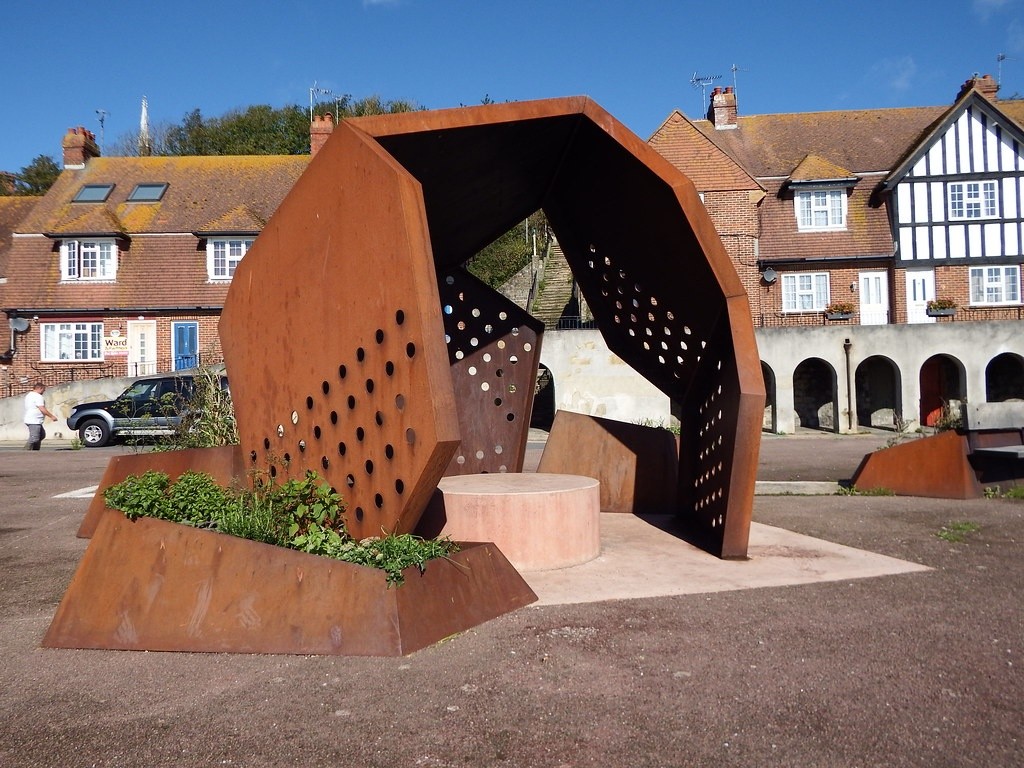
825,302,856,319
926,299,959,316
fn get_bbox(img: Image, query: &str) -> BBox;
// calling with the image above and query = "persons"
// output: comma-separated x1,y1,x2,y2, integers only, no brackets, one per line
23,382,58,450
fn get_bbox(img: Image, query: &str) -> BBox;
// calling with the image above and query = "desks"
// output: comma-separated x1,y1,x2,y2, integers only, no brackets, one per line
412,472,601,573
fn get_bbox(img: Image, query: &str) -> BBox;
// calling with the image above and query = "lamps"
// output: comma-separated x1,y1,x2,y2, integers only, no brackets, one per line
852,281,857,291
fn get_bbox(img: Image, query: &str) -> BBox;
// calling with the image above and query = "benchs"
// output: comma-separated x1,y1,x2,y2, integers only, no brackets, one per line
963,402,1024,458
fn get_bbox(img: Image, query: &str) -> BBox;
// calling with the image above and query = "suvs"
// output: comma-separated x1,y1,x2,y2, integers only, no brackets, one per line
66,375,235,447
